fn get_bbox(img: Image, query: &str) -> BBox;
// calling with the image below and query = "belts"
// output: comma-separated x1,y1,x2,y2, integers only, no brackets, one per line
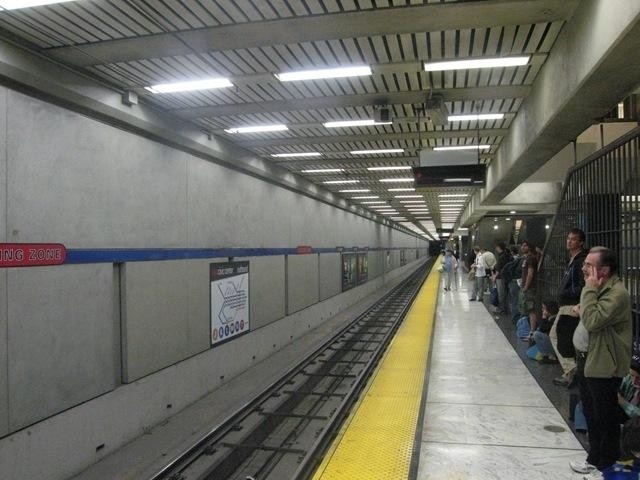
575,348,588,358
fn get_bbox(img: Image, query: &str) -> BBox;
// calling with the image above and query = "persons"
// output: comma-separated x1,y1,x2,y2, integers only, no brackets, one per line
521,284,559,364
437,249,459,292
549,229,639,480
468,238,558,326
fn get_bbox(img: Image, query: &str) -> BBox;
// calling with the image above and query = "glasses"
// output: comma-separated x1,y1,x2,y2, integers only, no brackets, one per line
632,354,640,363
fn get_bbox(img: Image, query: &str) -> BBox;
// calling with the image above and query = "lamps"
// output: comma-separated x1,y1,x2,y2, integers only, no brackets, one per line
424,89,451,129
371,101,392,123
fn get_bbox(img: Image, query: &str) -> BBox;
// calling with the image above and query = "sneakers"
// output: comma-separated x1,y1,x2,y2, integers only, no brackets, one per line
569,460,604,480
539,358,559,364
554,366,578,387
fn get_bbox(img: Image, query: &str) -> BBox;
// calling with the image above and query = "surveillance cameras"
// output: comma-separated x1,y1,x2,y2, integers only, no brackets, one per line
427,99,449,127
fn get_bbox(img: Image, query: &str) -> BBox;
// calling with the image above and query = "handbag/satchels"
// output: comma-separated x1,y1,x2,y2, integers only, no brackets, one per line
437,267,444,273
557,315,581,358
515,316,530,339
489,288,498,306
468,269,476,280
